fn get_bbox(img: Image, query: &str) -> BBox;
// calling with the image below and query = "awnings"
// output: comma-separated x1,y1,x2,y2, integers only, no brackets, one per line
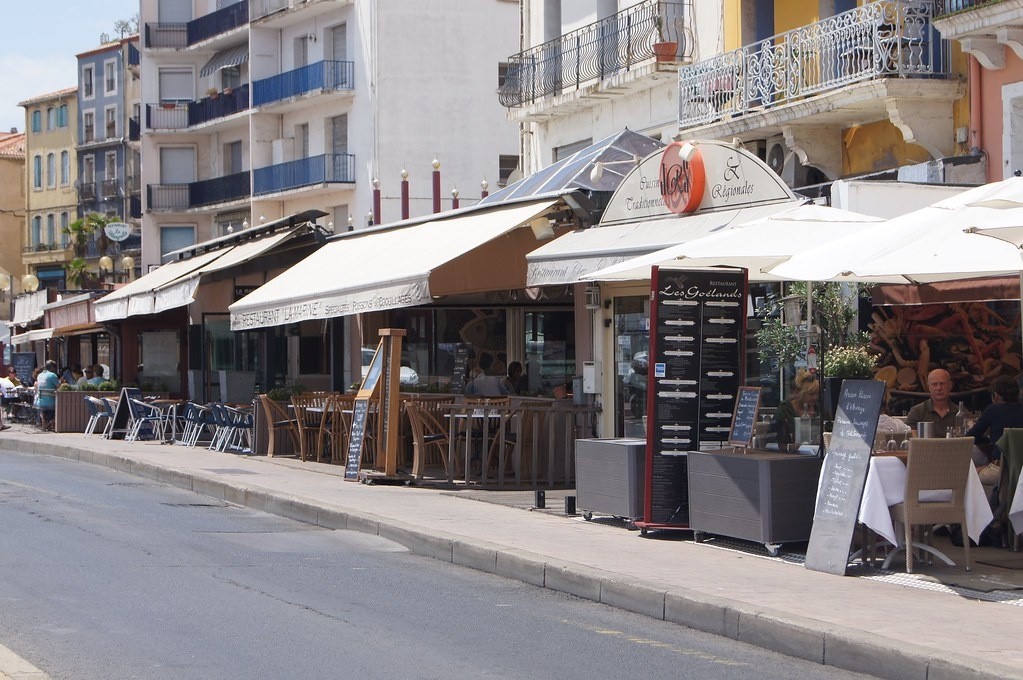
92,222,317,331
9,315,107,347
525,198,814,292
225,198,566,333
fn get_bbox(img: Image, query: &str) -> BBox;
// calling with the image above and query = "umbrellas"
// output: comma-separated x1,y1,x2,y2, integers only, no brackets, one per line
576,204,892,383
758,173,1023,284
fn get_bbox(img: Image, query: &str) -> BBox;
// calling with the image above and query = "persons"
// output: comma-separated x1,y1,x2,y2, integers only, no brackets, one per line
761,366,824,454
0,376,20,422
848,407,912,555
3,367,25,417
963,370,1023,544
464,348,552,466
30,357,120,434
904,367,974,449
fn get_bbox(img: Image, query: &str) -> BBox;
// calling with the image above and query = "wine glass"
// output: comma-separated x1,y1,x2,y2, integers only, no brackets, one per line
885,428,898,453
900,427,910,453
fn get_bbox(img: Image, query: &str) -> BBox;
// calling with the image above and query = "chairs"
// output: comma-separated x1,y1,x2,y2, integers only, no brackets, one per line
973,426,1023,550
870,432,888,454
0,368,254,454
257,376,557,488
883,435,977,575
822,432,832,453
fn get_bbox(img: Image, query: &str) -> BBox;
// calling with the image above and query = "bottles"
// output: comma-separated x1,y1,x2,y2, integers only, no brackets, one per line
956,401,967,436
799,402,811,444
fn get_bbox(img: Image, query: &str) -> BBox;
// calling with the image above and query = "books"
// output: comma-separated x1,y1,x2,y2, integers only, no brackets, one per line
794,443,822,456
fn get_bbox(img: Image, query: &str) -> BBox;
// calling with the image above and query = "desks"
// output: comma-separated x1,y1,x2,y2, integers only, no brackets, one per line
859,451,910,568
